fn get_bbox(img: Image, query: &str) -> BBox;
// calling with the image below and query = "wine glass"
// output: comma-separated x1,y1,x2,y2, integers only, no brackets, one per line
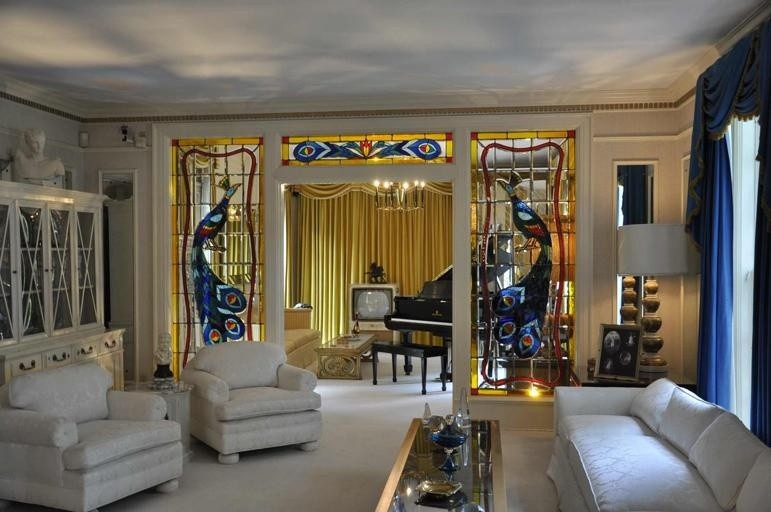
434,416,467,471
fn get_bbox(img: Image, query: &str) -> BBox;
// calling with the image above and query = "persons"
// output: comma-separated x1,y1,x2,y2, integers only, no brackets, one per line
12,126,65,186
154,333,173,365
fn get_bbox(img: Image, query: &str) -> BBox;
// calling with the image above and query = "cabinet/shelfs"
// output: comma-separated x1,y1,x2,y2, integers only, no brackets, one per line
498,355,574,389
128,380,195,464
0,181,126,391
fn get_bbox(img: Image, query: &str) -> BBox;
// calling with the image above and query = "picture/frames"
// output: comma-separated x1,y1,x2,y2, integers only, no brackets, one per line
592,324,643,381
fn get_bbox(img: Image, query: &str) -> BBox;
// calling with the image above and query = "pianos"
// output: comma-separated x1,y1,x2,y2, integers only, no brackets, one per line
383,234,513,383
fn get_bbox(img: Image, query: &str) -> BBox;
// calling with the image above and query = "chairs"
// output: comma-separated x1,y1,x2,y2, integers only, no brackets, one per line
0,362,183,512
178,341,321,464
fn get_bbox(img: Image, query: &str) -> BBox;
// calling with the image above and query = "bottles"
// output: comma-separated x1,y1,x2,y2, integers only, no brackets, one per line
456,384,472,430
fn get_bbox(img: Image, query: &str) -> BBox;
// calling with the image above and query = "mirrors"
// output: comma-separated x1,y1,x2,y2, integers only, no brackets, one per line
98,167,139,386
612,158,659,325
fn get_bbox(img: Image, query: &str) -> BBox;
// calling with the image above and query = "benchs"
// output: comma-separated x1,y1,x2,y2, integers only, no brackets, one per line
369,341,448,395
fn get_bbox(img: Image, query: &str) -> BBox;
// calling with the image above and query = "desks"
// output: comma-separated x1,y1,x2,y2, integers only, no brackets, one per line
350,320,402,343
570,365,698,388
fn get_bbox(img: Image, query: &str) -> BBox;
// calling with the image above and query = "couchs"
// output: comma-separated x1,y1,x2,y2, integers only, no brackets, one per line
545,377,771,512
282,308,321,369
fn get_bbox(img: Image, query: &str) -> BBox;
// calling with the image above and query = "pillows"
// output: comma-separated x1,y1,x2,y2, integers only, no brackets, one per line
630,377,679,434
659,387,726,458
689,411,767,511
734,447,771,512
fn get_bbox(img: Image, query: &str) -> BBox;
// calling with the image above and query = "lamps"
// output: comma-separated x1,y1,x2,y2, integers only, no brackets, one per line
373,179,427,214
617,224,698,372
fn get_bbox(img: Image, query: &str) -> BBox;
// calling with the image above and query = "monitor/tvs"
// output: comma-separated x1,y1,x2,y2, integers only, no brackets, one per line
348,284,398,331
